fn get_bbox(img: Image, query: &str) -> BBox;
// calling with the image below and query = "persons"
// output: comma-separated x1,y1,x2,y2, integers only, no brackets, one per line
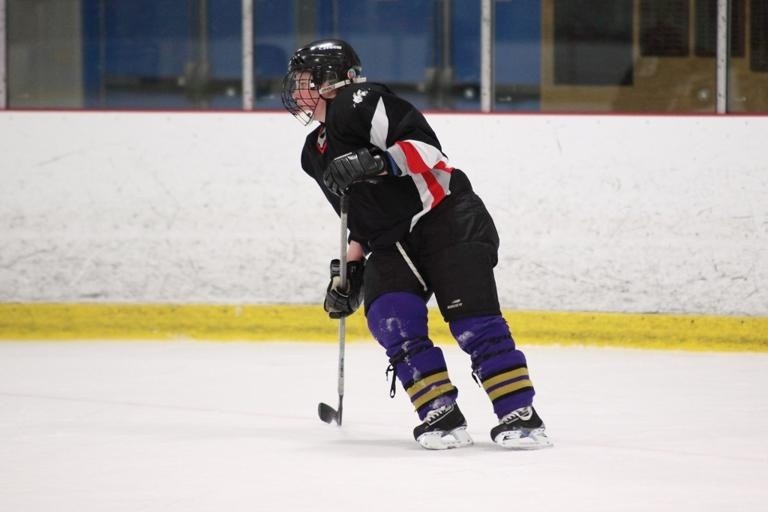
281,39,553,449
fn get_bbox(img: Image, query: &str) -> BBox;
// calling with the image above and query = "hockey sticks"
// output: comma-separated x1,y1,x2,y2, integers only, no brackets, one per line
318,196,348,425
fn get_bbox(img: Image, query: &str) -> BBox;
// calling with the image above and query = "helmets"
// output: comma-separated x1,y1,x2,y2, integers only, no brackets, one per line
280,38,367,128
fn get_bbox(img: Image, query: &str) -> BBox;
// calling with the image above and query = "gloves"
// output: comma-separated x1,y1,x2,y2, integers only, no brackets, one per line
322,254,368,320
322,144,386,198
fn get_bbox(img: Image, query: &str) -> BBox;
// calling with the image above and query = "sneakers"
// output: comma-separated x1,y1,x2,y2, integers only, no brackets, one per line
413,399,466,438
486,406,547,440
